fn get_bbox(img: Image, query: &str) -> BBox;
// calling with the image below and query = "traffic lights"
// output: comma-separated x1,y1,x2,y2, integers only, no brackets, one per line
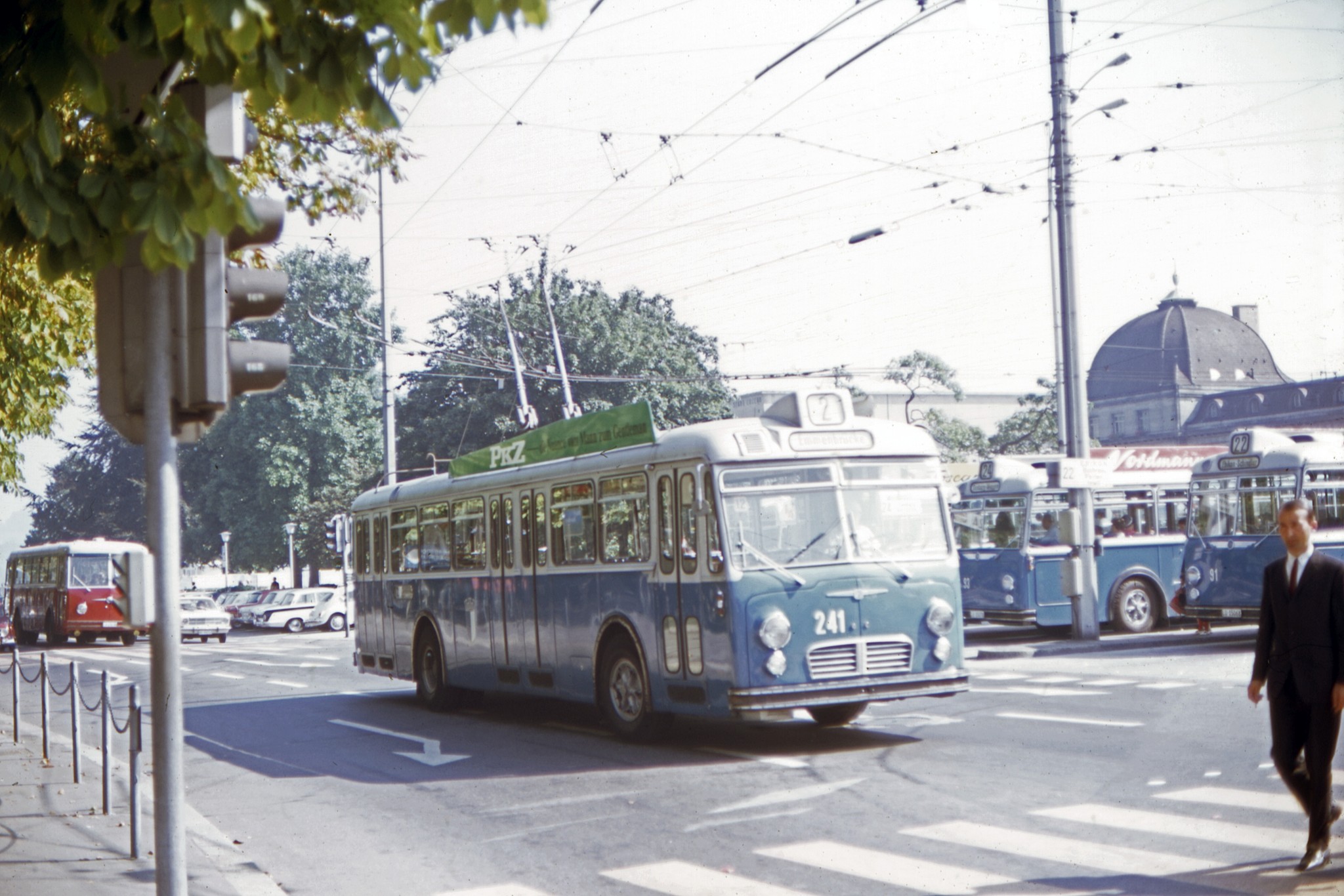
174,82,290,416
323,518,341,553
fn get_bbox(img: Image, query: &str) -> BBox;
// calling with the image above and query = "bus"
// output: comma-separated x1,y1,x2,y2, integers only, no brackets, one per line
350,250,972,745
4,537,154,647
951,427,1343,634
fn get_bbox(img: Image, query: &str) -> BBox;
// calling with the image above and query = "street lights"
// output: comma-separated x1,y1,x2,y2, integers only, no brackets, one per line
282,523,298,588
1050,54,1126,451
219,532,232,587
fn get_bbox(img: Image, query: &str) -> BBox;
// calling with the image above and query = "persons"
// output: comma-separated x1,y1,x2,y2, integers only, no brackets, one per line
826,525,880,558
91,572,105,585
1178,518,1187,528
1248,501,1344,872
192,583,196,589
1035,515,1061,545
996,512,1018,548
197,601,205,609
270,577,279,590
1111,518,1125,539
239,581,243,585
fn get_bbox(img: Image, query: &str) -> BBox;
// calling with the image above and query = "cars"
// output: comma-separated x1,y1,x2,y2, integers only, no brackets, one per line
180,588,354,643
0,603,16,651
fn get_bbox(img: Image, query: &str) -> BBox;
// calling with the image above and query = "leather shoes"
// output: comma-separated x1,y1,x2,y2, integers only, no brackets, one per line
1327,799,1342,826
1297,848,1329,871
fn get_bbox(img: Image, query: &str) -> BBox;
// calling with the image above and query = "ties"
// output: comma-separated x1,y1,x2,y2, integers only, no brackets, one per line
1289,557,1300,595
